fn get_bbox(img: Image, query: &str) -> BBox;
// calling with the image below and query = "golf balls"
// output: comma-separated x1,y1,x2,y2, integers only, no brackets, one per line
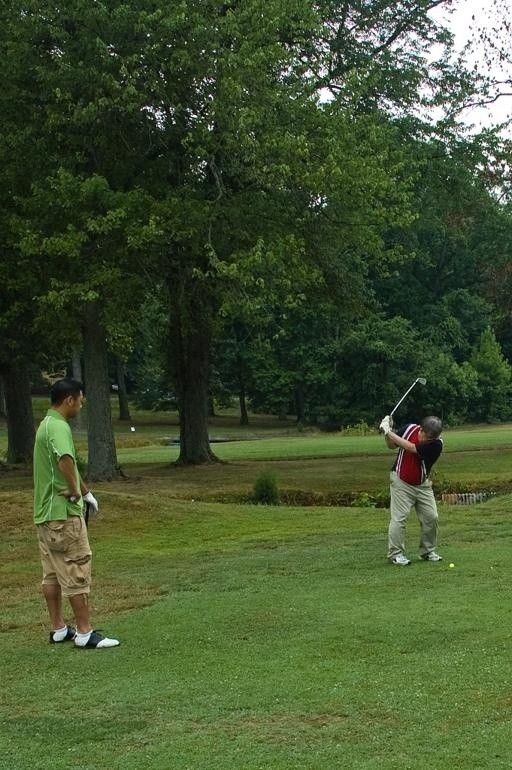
449,563,453,567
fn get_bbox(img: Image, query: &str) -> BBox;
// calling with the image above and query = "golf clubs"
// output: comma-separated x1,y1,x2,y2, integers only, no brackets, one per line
378,377,427,435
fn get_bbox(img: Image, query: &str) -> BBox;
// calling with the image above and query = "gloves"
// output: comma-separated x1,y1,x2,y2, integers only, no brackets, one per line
83,491,100,513
378,421,392,435
382,415,391,424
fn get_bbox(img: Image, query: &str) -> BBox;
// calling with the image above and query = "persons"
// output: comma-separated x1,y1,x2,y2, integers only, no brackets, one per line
378,415,442,566
31,376,122,649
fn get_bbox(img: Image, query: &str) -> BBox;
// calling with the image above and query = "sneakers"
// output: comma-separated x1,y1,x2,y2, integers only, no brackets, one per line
417,551,442,562
74,628,121,648
391,554,411,566
49,625,77,643
